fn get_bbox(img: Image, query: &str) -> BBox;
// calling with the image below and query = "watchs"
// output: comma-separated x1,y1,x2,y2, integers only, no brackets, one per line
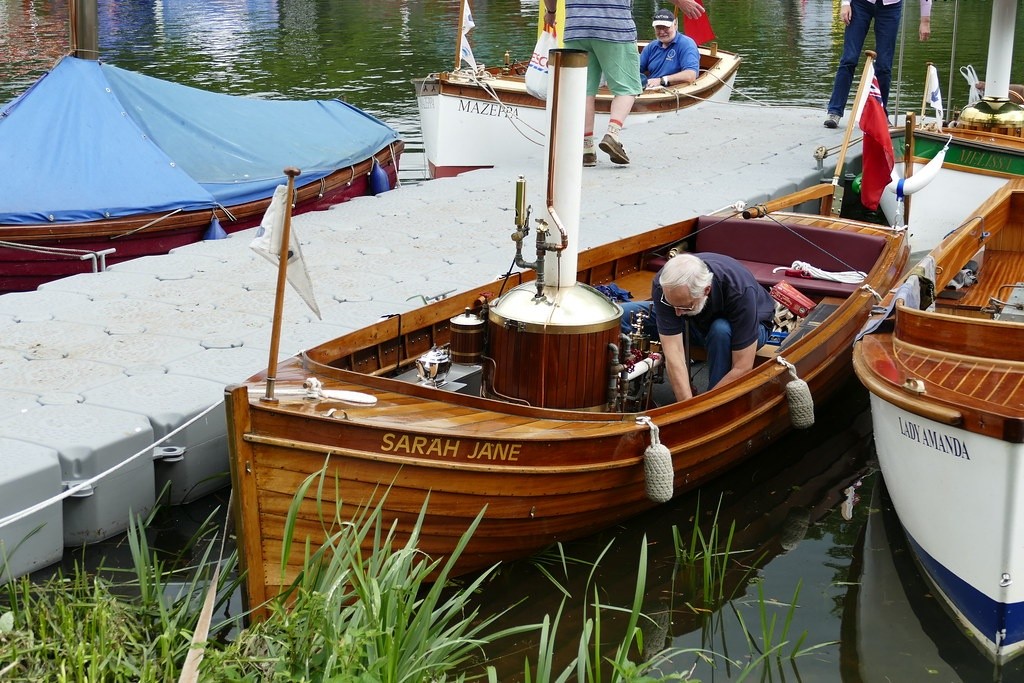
545,6,556,14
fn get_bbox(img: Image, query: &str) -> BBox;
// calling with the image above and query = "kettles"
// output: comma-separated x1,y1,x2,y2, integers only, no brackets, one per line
414,343,452,381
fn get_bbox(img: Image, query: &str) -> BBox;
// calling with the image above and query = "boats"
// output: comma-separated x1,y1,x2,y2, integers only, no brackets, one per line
0,55,405,292
859,110,1024,257
413,40,744,178
223,201,911,629
850,178,1023,683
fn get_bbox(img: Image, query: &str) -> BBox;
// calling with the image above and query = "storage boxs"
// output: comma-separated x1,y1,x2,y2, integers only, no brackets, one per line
768,280,817,319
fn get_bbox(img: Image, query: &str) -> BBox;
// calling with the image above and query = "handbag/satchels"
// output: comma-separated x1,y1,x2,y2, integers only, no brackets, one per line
525,21,560,100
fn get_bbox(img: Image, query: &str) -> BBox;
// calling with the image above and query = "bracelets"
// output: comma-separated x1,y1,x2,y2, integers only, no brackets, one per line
660,75,670,87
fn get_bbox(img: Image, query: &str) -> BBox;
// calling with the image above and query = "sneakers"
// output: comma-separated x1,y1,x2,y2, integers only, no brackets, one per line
599,134,630,163
583,151,597,166
823,114,841,128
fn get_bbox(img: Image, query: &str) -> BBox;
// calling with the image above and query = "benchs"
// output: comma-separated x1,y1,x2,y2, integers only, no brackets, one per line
642,214,884,299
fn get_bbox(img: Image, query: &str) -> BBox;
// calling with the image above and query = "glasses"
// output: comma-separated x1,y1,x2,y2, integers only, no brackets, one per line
661,294,697,311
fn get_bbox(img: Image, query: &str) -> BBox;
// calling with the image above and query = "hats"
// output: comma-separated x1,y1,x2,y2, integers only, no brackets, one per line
652,9,674,27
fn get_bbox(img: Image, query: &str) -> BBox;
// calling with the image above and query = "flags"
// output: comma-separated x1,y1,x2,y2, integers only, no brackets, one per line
855,62,895,211
926,65,943,133
683,0,715,46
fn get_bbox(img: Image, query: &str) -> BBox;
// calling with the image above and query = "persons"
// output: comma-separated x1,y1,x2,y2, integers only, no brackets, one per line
640,8,700,88
652,252,769,408
824,0,932,128
526,0,706,166
